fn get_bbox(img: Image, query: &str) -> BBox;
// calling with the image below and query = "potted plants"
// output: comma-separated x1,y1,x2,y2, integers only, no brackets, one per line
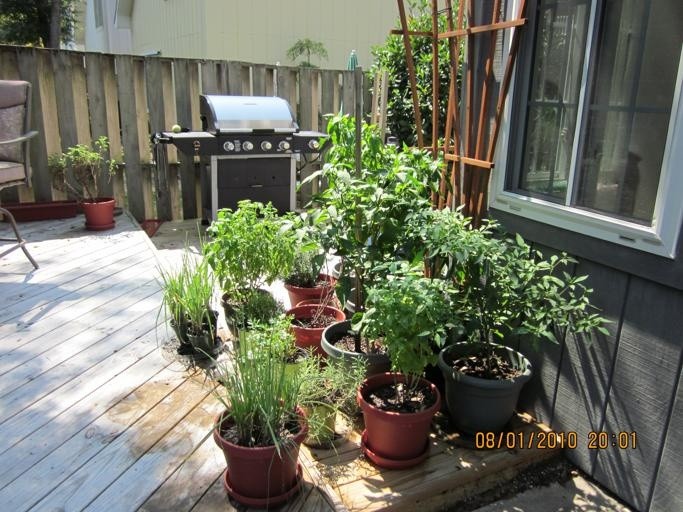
292,348,369,451
343,264,391,314
280,205,338,307
256,316,308,376
315,113,448,282
421,210,610,444
408,198,490,346
302,173,434,412
201,329,339,506
284,268,343,360
184,242,219,338
52,136,130,228
205,194,298,338
159,262,192,356
356,275,450,469
176,275,216,357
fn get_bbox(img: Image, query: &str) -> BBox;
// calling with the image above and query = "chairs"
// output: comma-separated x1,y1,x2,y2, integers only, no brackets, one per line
0,72,38,276
